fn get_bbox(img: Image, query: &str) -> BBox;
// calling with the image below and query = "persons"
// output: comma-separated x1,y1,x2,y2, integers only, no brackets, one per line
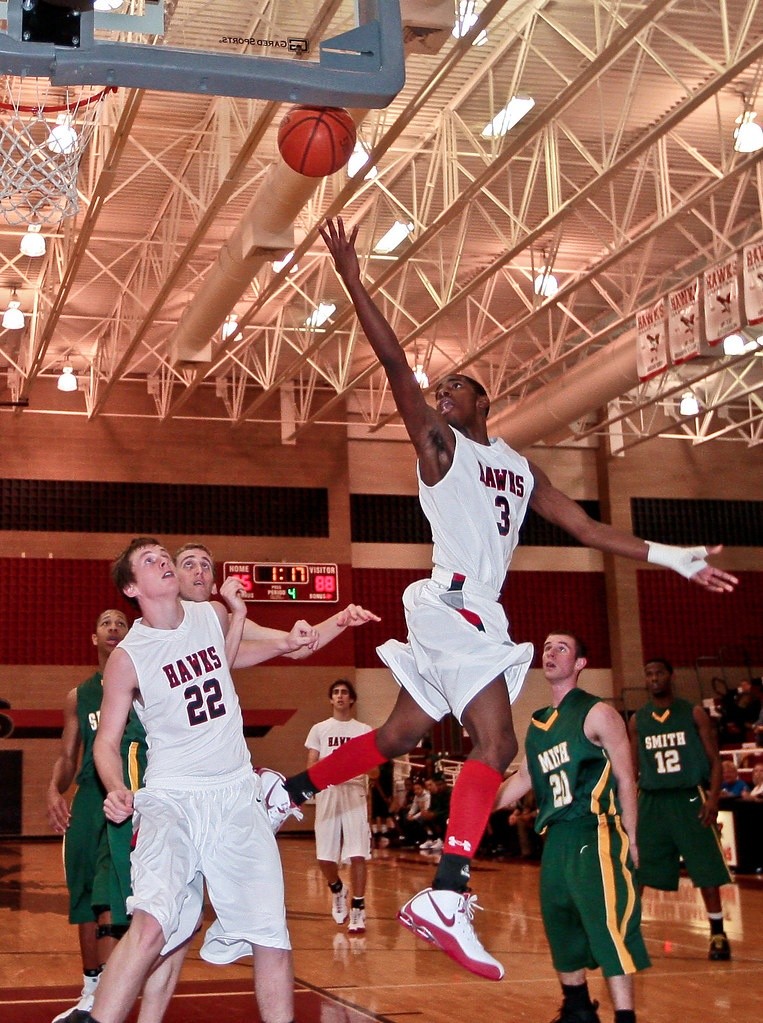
397,773,451,850
257,213,739,983
45,536,381,1023
626,657,734,961
496,629,638,1023
490,792,542,862
369,753,411,838
708,673,763,801
304,679,373,934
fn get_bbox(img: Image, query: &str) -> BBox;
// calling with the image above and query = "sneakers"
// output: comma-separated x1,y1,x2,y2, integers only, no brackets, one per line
259,766,304,836
52,987,98,1023
332,933,350,975
346,897,367,932
331,883,349,924
346,933,367,957
708,933,732,959
397,886,505,982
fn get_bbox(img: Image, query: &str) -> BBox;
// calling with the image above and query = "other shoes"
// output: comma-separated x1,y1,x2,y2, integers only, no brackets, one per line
550,999,604,1023
419,840,434,849
430,838,443,850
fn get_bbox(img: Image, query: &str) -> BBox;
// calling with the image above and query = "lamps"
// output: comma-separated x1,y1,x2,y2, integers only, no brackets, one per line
2,289,24,329
57,354,78,391
20,214,46,257
48,113,80,153
734,94,762,153
372,219,415,254
481,91,535,140
534,257,557,296
680,375,699,415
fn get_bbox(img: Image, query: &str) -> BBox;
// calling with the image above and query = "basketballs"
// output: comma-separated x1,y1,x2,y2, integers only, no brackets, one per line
277,103,358,178
